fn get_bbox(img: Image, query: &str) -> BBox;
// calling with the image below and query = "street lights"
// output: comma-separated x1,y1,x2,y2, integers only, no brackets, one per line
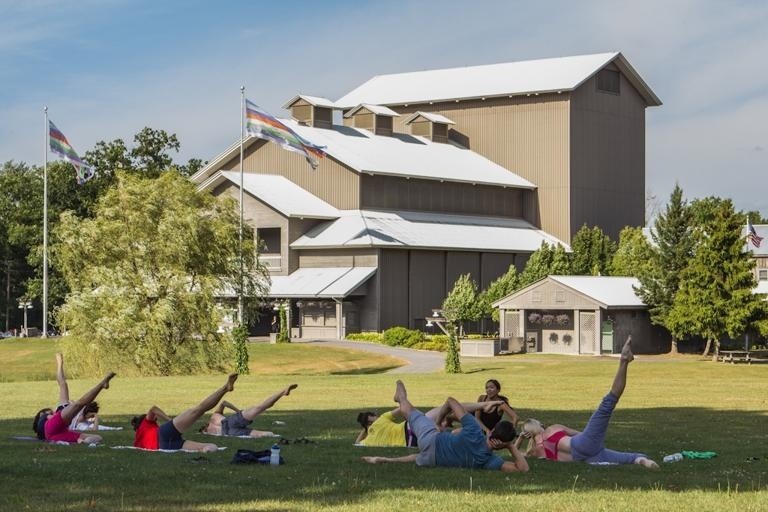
18,298,34,336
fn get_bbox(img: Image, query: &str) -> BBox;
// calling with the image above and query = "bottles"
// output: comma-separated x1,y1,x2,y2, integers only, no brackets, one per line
662,452,685,464
271,420,285,427
269,442,281,467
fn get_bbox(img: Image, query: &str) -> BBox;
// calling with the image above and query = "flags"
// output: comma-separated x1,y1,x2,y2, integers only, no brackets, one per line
748,221,764,248
243,97,330,172
45,117,97,183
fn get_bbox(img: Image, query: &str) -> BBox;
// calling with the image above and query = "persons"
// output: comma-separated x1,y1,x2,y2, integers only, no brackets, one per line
131,372,238,454
439,379,521,435
32,370,116,447
354,400,508,449
198,381,298,440
54,352,101,433
361,377,531,478
511,334,661,471
